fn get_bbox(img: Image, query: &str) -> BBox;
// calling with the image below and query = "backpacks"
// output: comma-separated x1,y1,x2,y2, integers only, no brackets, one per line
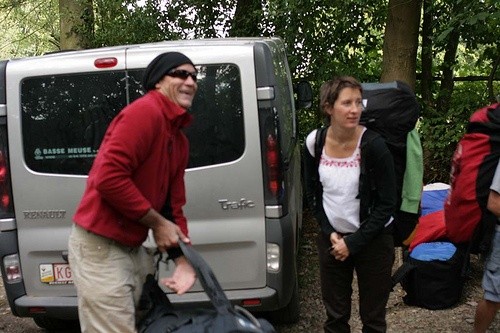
358,80,424,247
389,182,471,310
444,102,500,255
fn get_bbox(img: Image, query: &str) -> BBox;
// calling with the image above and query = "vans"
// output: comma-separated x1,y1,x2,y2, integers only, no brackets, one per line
0,36,313,328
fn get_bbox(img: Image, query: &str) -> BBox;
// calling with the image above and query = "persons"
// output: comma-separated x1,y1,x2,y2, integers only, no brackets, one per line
67,52,196,333
473,160,500,333
300,75,399,333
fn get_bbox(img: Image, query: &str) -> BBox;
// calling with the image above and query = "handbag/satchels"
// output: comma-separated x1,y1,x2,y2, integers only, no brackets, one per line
135,241,275,333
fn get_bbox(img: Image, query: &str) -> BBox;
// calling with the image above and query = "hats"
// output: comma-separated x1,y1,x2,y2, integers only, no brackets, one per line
141,52,195,90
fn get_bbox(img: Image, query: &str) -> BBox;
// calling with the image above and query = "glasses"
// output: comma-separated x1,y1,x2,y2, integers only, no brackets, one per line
166,69,197,82
